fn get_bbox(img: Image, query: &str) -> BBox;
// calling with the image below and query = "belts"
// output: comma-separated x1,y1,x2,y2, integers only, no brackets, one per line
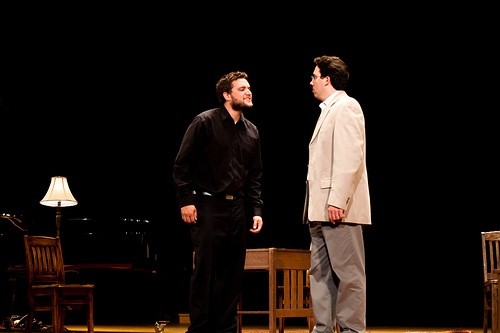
192,190,240,200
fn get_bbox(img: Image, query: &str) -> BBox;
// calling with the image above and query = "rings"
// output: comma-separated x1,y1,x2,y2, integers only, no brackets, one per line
335,215,340,218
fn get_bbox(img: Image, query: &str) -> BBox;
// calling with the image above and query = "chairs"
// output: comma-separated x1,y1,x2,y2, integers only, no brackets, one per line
480,230,500,333
278,250,341,333
23,235,95,333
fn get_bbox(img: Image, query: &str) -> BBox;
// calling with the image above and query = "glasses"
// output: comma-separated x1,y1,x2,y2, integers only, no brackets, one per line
311,76,321,81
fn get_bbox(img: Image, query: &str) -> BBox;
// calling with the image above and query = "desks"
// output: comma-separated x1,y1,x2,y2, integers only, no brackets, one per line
193,247,316,333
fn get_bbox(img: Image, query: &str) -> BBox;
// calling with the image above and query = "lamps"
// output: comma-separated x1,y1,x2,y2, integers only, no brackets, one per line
39,176,79,236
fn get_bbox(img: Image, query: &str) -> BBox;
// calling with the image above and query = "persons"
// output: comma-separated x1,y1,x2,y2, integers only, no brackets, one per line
302,55,372,333
173,71,263,333
